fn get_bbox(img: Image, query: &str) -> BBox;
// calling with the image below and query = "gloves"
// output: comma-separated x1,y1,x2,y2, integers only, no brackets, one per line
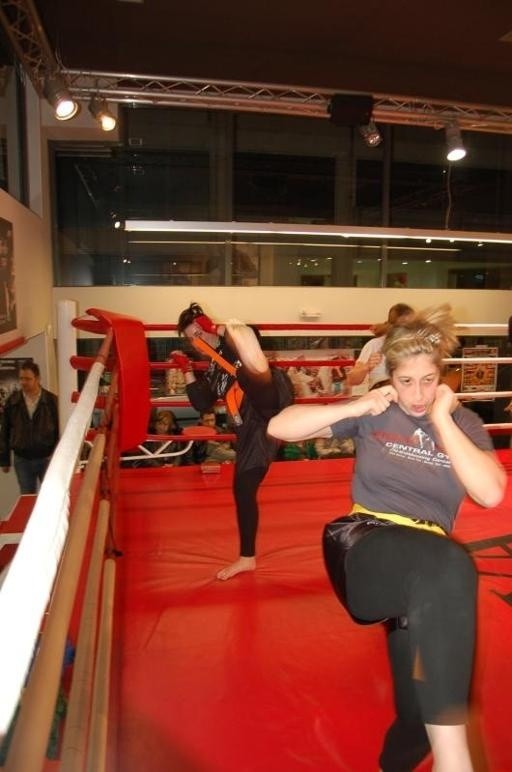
197,314,220,339
173,351,192,376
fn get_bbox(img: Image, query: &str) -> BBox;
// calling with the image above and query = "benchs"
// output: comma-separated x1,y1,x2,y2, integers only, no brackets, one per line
1,492,41,589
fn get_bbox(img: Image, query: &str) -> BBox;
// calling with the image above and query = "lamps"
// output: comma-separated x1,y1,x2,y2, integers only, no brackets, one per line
39,77,119,134
356,113,467,166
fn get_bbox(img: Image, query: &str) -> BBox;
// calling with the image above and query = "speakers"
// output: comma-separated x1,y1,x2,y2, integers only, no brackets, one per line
331,92,374,128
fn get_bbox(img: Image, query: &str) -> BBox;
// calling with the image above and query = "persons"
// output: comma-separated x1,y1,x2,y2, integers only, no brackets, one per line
345,305,416,392
266,301,508,770
84,350,363,466
0,361,59,494
169,301,295,581
